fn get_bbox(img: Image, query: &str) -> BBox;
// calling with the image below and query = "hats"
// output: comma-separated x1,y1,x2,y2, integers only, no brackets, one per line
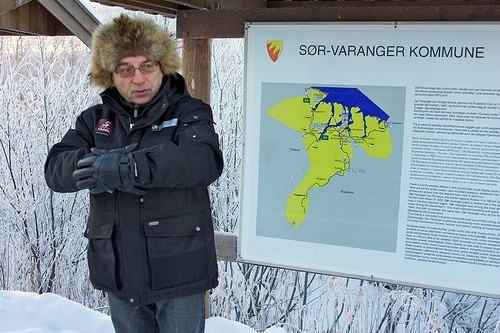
85,13,182,87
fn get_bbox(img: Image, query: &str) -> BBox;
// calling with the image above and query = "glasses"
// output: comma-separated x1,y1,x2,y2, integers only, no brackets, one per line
114,60,158,78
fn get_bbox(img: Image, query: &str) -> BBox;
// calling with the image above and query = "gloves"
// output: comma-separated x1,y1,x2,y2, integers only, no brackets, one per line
73,142,146,196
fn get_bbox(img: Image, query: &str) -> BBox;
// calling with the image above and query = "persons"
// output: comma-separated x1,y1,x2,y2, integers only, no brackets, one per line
44,12,224,333
